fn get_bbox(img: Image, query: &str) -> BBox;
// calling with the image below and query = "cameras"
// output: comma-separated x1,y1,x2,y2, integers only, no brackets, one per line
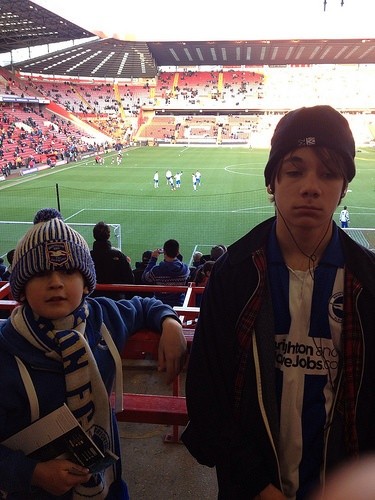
157,248,163,254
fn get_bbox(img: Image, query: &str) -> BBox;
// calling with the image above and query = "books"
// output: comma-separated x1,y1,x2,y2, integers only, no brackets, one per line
0,402,105,500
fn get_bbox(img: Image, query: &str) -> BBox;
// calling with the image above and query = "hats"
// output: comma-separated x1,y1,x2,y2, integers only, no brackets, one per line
263,103,356,187
9,210,98,298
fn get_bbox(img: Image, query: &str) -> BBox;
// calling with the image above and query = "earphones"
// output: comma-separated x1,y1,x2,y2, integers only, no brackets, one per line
341,189,347,197
270,184,274,193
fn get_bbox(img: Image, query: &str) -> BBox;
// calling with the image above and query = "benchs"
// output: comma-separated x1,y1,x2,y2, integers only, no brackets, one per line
0,66,264,174
0,280,206,445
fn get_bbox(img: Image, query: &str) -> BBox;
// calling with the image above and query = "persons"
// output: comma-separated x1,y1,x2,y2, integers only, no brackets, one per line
0,208,187,500
0,68,350,308
179,105,375,500
